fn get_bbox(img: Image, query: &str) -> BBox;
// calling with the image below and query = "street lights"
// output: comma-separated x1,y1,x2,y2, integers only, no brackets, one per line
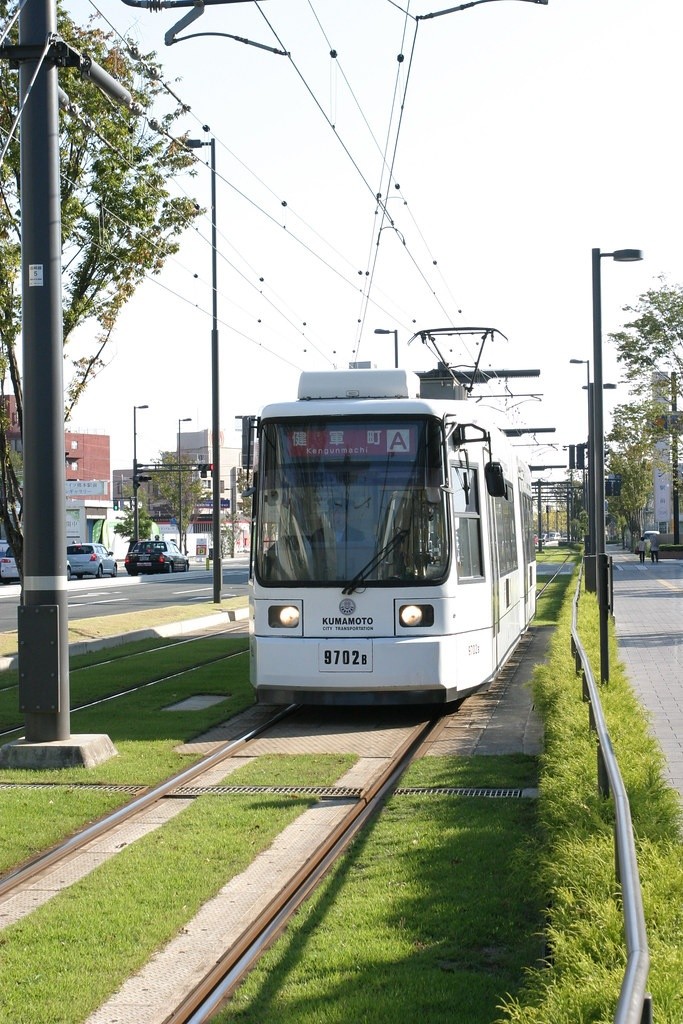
567,360,595,593
589,240,642,684
179,134,227,606
134,405,151,541
179,416,191,553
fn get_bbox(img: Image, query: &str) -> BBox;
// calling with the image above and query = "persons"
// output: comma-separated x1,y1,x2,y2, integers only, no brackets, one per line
637,537,646,563
309,494,367,544
649,533,659,563
155,534,160,540
534,534,538,545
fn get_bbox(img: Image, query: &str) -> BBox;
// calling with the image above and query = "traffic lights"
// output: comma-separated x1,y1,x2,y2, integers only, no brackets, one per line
207,464,213,471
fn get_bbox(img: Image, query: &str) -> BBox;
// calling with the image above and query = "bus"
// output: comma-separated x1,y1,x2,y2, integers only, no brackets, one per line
229,363,539,715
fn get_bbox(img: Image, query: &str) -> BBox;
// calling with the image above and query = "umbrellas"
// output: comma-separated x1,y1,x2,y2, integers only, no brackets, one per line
644,530,661,536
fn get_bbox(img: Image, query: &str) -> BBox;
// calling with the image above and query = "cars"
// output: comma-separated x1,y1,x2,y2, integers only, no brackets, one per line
66,540,118,578
125,540,189,576
1,542,71,586
